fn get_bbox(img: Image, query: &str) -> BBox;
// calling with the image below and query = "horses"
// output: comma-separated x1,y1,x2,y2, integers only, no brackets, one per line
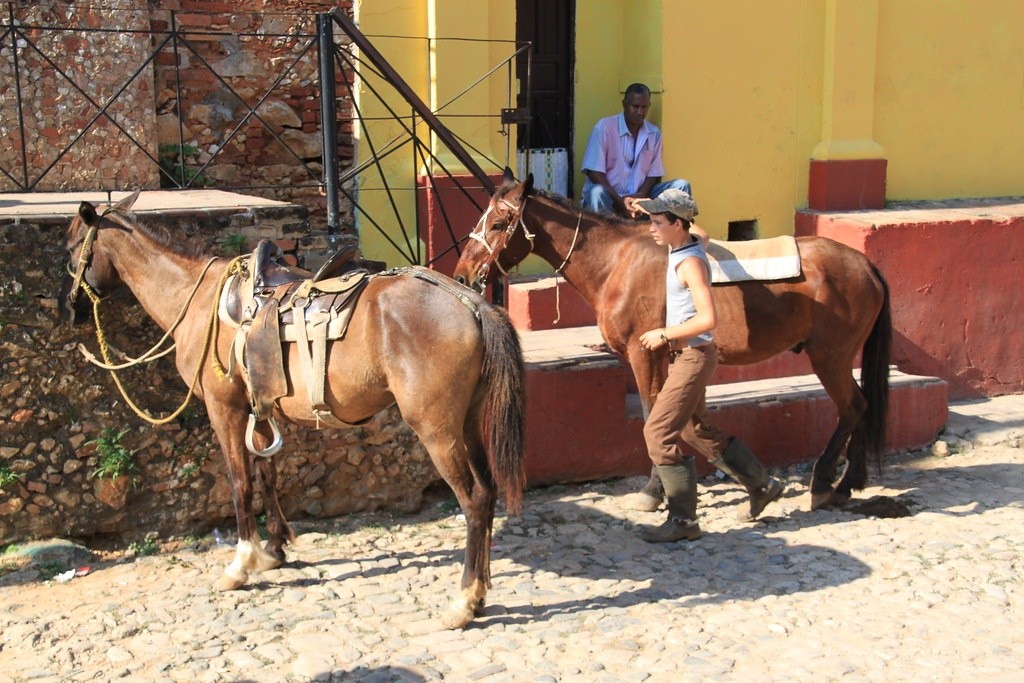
61,190,523,630
454,166,895,514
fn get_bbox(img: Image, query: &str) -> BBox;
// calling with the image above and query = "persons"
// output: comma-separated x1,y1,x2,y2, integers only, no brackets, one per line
580,83,691,221
631,189,784,543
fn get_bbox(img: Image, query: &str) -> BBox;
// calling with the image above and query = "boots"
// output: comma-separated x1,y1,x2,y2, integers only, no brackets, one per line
640,455,703,542
707,436,785,522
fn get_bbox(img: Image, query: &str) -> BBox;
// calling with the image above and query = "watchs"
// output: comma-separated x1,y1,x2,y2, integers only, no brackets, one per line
660,328,668,344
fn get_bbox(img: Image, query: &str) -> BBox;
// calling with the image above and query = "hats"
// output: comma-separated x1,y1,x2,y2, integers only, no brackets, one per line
636,189,695,224
